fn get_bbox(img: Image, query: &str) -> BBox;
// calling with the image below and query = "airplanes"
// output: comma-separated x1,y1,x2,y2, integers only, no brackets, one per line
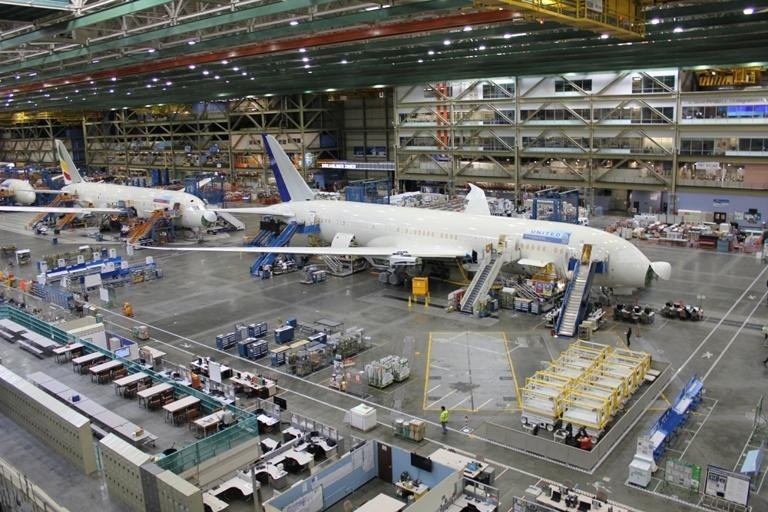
128,132,672,290
0,139,218,245
0,177,38,206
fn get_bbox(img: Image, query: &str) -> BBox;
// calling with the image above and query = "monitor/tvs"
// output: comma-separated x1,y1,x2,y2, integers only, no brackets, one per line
411,453,432,472
114,346,130,359
274,396,286,410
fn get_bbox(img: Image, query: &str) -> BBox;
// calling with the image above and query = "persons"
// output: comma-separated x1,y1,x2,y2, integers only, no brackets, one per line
440,406,452,437
625,328,632,350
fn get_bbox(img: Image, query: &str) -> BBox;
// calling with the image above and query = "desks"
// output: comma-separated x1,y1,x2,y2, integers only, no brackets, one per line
229,371,276,397
426,448,490,478
202,414,338,512
395,479,429,500
353,493,407,512
52,342,231,441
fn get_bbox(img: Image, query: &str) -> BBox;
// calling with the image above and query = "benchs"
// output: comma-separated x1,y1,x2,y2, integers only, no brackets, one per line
658,236,689,247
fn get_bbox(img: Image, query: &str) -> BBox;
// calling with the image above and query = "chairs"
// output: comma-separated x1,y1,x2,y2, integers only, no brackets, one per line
343,499,355,512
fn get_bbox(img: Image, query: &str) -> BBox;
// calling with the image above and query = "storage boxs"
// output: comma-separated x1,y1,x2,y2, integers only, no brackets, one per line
408,420,425,441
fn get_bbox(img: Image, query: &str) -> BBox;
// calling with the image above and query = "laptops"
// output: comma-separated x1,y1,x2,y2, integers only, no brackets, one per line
551,491,561,502
